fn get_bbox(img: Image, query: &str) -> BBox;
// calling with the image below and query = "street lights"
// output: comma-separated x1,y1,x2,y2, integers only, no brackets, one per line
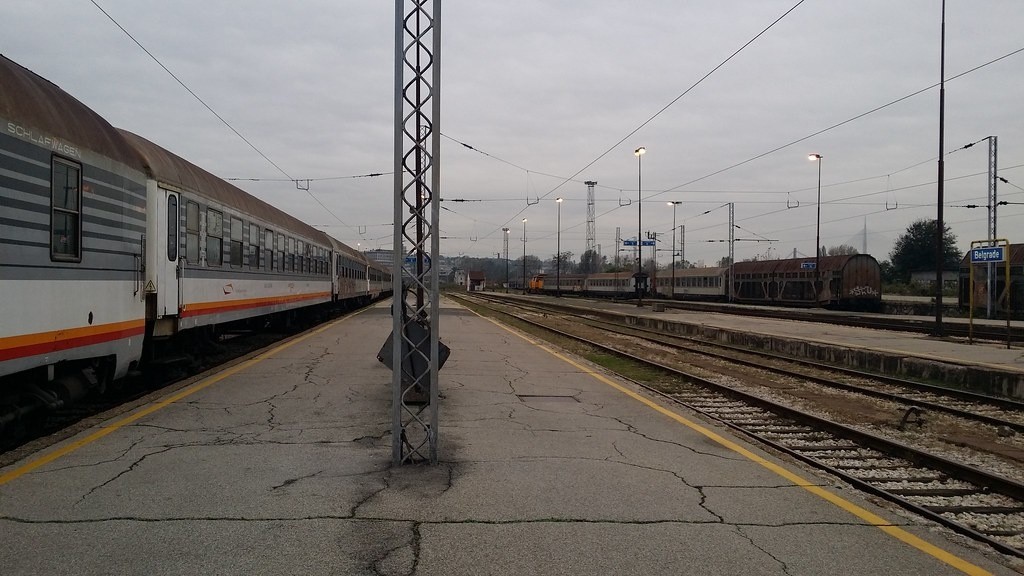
634,146,647,307
556,197,564,299
521,217,528,295
667,201,683,299
807,152,824,308
505,228,512,294
357,243,360,251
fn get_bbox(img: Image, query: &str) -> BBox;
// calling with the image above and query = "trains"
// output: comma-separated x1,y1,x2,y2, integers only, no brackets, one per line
525,252,884,311
1,50,417,418
957,242,1024,315
